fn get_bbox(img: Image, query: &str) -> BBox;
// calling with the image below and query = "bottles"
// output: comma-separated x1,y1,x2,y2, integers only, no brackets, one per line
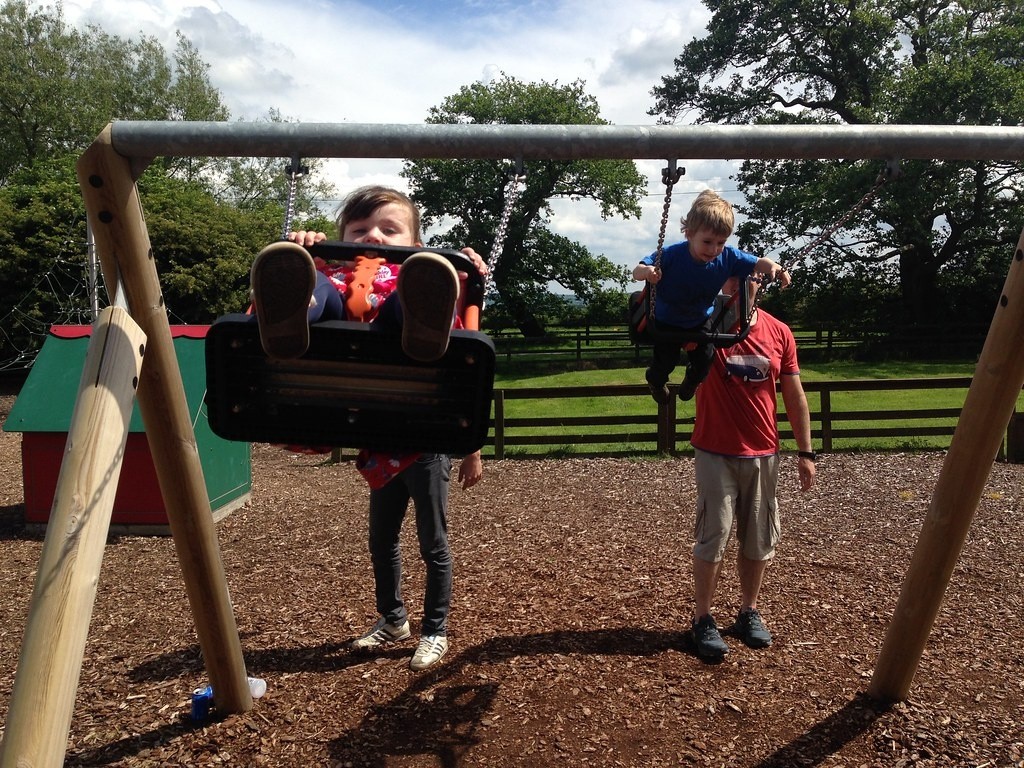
247,677,267,698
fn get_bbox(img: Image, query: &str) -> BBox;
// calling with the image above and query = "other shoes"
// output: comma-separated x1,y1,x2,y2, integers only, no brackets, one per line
251,242,318,358
396,253,461,362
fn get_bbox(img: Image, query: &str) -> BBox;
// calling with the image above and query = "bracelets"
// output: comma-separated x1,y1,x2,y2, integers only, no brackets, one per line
798,451,817,460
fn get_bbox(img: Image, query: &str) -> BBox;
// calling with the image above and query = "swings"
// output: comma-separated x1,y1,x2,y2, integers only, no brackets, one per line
628,175,885,347
203,172,521,456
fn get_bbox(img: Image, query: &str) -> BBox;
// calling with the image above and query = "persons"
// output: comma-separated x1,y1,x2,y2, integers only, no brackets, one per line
249,184,483,672
634,188,792,406
635,248,820,658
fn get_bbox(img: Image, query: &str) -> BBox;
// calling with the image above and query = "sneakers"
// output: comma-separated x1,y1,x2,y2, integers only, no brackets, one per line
738,608,771,646
692,614,730,660
352,616,411,650
679,377,698,401
648,382,671,404
410,634,448,670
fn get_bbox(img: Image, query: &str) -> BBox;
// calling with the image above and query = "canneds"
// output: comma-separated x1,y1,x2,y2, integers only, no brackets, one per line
192,682,213,723
